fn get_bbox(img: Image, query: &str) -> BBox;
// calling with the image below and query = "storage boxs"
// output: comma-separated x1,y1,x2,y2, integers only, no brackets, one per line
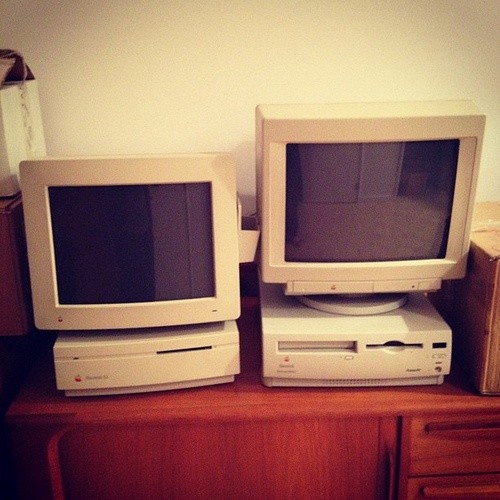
451,229,500,396
0,48,46,338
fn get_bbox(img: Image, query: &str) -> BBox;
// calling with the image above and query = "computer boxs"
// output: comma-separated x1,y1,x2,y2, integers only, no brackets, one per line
53,319,241,399
259,281,453,388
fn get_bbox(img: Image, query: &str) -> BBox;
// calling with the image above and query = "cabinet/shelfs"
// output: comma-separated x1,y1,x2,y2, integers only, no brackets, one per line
8,295,500,500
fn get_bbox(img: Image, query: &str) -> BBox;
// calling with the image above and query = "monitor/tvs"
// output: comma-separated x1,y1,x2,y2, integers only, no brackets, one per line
254,101,487,313
16,151,242,330
